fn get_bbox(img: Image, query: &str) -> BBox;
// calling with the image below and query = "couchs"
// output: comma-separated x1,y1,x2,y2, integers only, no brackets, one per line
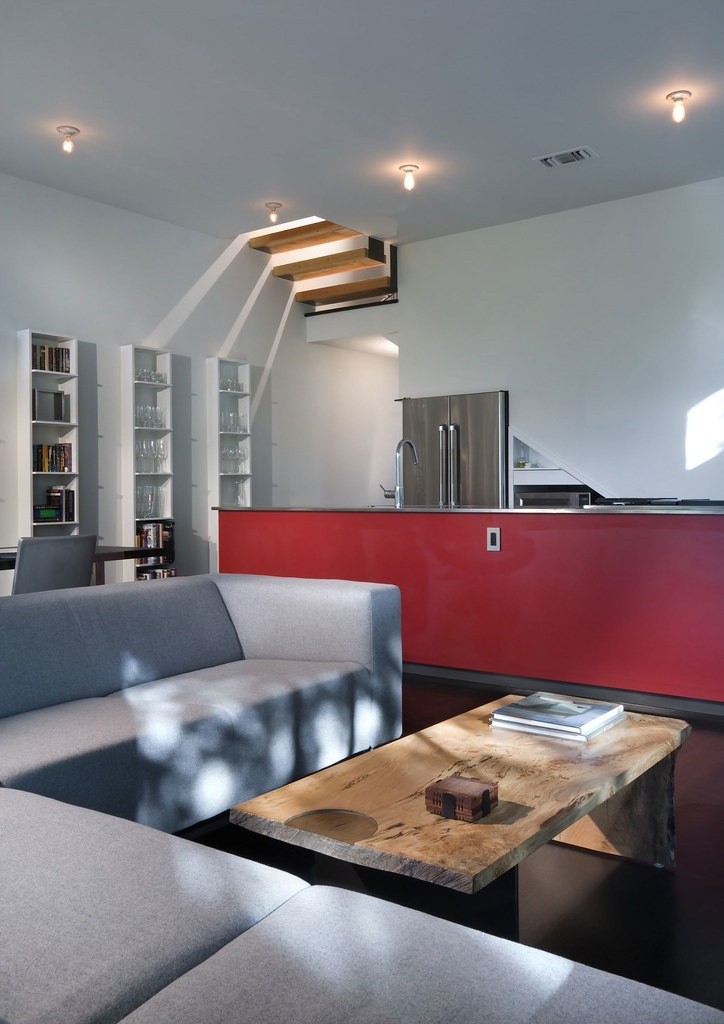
0,575,722,1024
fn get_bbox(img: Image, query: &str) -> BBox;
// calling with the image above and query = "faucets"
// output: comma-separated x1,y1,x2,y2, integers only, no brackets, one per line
378,438,418,506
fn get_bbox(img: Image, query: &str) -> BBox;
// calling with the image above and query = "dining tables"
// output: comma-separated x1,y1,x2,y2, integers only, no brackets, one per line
0,546,166,586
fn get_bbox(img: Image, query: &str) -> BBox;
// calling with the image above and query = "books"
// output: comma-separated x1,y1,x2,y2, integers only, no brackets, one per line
489,691,627,741
33,443,72,472
32,345,70,372
34,485,74,522
135,521,176,581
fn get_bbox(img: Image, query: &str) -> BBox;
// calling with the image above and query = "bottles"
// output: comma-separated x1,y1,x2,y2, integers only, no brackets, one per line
518,455,525,467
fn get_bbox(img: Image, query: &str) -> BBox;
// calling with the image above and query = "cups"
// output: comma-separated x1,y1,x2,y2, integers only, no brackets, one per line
525,463,530,468
532,462,537,467
221,410,248,432
233,480,245,507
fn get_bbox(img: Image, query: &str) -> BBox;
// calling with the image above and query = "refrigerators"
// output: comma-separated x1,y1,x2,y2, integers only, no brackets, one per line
401,390,510,508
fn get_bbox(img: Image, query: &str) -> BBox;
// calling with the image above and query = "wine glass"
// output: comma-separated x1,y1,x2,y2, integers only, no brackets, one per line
221,375,240,390
135,405,163,427
222,447,247,474
136,484,161,517
136,439,167,474
136,366,163,383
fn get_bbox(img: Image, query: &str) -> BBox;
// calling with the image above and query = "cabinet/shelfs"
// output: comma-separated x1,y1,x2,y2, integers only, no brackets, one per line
206,355,252,507
119,346,174,582
17,328,80,537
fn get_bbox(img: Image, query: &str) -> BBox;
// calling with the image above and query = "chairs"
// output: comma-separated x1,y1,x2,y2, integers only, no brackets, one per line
10,536,97,595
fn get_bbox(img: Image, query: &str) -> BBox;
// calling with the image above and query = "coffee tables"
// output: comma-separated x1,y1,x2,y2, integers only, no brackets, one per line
229,690,692,943
